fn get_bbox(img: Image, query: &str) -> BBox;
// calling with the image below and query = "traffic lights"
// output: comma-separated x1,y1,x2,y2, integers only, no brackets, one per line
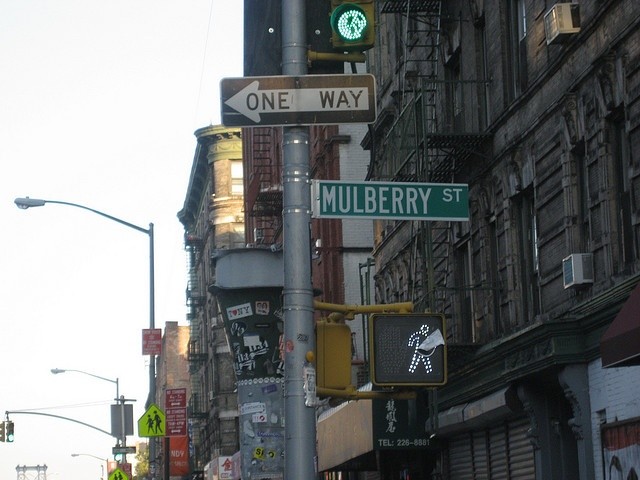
6,423,14,442
0,423,5,441
367,305,452,393
330,0,376,49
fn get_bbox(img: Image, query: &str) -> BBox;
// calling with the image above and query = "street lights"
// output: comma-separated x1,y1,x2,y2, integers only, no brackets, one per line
71,454,110,480
50,368,118,447
14,197,157,480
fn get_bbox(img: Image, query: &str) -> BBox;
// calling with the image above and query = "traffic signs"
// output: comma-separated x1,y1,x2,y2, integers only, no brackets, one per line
220,73,377,127
311,179,471,222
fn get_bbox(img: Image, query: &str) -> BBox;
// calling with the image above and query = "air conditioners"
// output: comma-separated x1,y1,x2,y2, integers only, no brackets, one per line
562,252,595,290
543,2,582,46
